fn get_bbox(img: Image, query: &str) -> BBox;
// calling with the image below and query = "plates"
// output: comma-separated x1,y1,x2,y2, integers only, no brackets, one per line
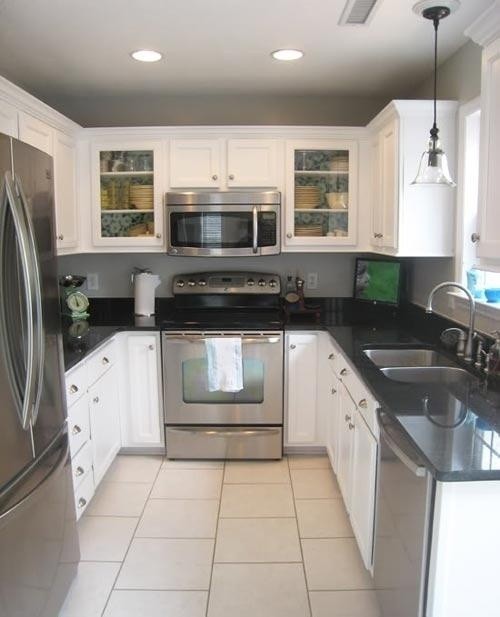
294,224,323,236
294,185,321,209
329,155,349,171
129,184,153,209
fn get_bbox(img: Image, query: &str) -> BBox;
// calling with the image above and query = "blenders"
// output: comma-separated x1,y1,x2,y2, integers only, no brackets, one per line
128,271,161,316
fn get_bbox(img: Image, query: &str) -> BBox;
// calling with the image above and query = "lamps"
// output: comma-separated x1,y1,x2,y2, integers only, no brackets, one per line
407,0,462,190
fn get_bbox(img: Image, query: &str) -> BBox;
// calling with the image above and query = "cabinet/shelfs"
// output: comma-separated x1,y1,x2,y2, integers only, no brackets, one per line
77,126,168,254
117,330,166,450
282,125,367,254
336,355,383,574
465,2,500,271
320,337,341,476
169,124,284,196
82,337,122,486
284,332,330,446
1,76,19,143
65,361,97,523
367,99,459,261
19,89,80,255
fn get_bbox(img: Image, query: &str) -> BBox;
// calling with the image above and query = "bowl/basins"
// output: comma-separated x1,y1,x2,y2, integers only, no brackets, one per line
484,288,500,302
325,192,348,209
101,180,134,209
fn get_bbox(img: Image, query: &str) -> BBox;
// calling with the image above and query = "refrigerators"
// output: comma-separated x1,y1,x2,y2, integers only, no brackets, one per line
0,130,81,617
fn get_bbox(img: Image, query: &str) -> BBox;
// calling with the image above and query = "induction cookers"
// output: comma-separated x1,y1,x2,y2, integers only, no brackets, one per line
162,271,281,330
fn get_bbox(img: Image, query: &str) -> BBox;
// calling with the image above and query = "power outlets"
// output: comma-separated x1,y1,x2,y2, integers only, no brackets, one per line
86,273,99,290
307,273,319,291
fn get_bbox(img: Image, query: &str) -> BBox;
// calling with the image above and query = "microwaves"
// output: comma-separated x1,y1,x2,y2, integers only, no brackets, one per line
164,190,281,258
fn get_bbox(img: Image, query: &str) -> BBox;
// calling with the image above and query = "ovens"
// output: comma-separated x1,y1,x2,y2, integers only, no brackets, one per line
161,330,284,461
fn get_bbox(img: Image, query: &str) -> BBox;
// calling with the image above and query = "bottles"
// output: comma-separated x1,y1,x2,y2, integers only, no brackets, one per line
285,275,295,294
296,279,305,302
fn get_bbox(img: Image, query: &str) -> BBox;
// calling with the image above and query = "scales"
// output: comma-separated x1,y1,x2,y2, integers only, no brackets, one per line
58,274,90,320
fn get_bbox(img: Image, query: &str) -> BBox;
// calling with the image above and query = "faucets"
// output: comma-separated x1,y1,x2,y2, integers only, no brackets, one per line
428,280,476,360
421,385,470,426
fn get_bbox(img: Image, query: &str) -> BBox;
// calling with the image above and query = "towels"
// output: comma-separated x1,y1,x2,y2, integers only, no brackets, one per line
204,338,244,393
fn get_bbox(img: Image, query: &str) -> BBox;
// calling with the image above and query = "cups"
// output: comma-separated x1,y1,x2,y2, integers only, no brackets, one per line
124,158,150,171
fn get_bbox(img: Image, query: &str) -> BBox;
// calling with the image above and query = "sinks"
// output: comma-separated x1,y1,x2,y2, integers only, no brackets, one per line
380,367,480,385
366,348,461,368
